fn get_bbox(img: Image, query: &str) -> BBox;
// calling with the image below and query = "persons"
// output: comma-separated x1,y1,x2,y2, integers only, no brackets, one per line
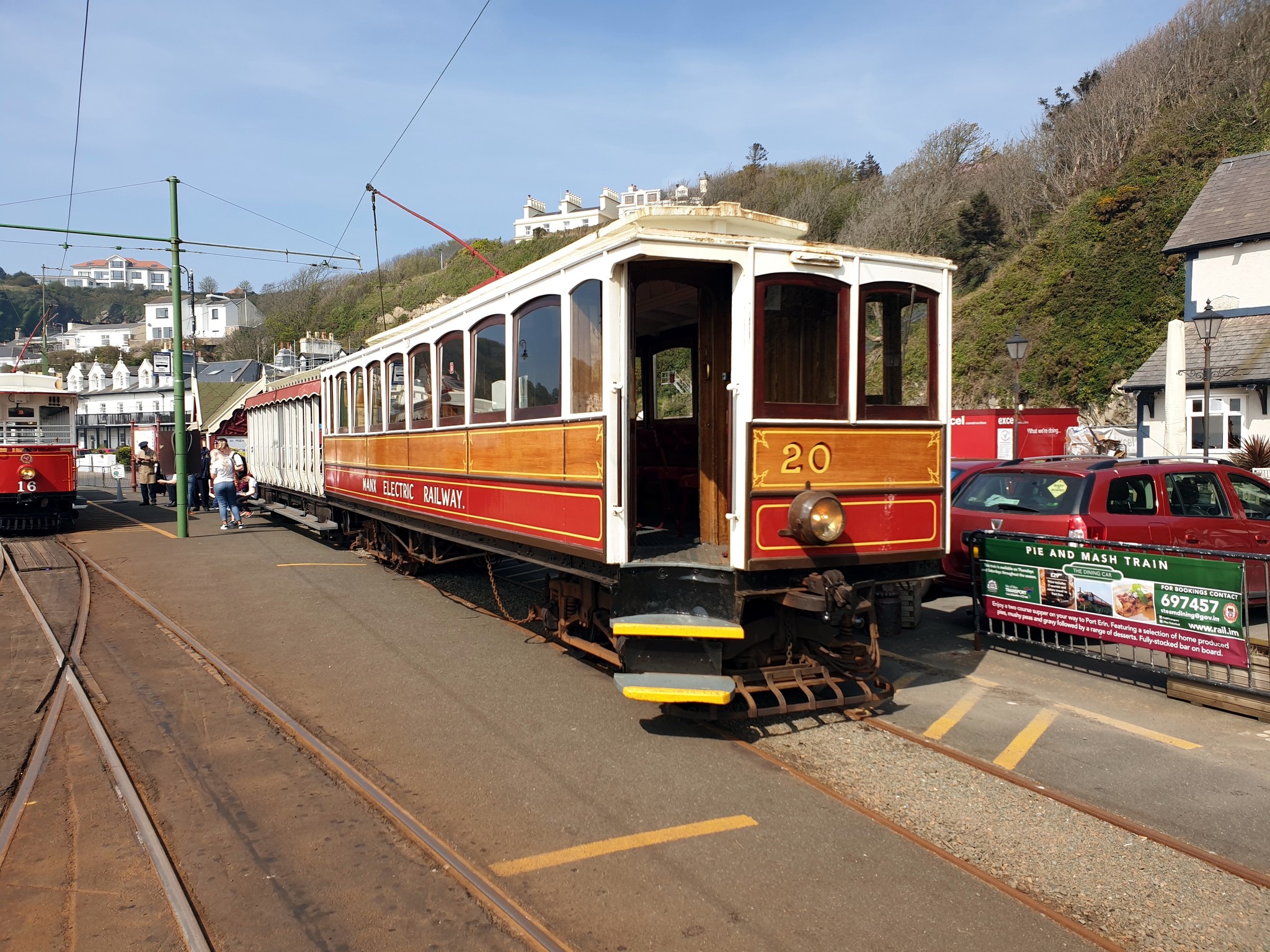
208,448,247,530
133,441,158,506
157,442,211,512
215,441,243,529
209,438,258,518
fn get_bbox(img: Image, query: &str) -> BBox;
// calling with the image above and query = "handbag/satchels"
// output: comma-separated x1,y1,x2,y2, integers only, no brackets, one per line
229,457,240,487
232,452,248,480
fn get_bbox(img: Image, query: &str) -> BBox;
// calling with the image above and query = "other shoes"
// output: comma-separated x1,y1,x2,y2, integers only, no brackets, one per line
188,511,196,514
139,503,149,506
209,505,216,509
192,508,200,511
164,501,172,507
152,502,156,505
205,508,209,511
167,501,177,507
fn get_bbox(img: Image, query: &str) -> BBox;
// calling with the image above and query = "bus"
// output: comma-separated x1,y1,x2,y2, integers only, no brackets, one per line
0,306,83,525
242,188,958,718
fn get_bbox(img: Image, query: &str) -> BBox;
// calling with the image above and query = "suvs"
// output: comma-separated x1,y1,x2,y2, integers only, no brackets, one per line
939,454,1270,640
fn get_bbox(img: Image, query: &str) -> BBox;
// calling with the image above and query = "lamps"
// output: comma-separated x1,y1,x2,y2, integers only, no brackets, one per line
519,340,529,360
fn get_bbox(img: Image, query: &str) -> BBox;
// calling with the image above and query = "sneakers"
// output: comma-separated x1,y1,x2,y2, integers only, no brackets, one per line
220,524,228,530
239,510,246,516
244,511,254,518
238,523,247,529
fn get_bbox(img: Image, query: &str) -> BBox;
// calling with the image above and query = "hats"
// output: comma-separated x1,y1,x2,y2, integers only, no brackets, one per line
138,441,148,450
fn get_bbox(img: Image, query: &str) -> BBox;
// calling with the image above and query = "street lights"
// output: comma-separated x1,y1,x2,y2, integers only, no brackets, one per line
1193,297,1225,467
174,264,197,380
1004,328,1031,459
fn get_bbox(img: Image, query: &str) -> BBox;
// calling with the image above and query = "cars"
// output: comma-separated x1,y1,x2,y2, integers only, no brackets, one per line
75,448,92,456
91,448,117,454
950,458,1042,516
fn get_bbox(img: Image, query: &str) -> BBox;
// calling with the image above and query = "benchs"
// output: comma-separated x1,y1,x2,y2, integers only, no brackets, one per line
248,498,338,530
